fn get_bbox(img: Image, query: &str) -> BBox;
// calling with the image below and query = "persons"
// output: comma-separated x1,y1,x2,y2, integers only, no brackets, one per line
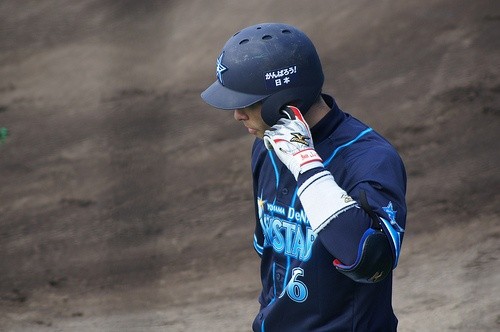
200,23,407,332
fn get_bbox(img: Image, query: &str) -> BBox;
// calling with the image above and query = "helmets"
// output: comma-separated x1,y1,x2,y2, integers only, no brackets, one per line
201,23,324,110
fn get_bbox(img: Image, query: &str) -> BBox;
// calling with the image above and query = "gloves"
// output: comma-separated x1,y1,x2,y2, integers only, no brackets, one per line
264,105,324,181
